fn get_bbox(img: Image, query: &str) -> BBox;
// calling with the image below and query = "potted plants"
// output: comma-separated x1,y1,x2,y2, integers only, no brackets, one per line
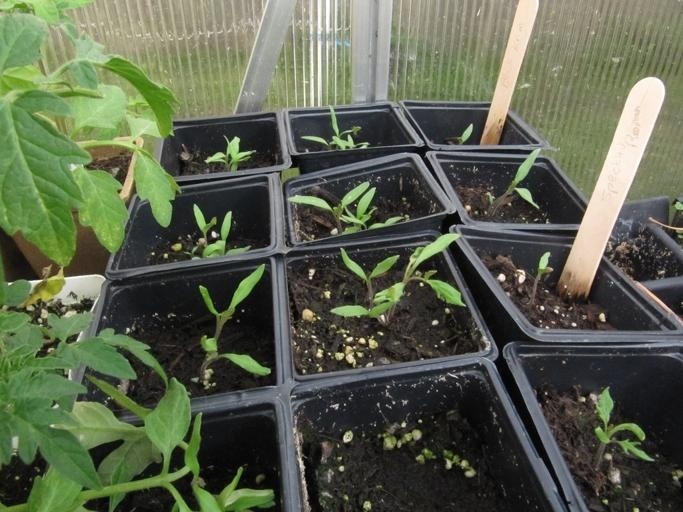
283,153,456,245
426,151,588,234
502,343,682,512
286,358,566,511
283,101,424,176
277,229,499,385
0,0,296,511
449,222,683,343
399,101,553,161
